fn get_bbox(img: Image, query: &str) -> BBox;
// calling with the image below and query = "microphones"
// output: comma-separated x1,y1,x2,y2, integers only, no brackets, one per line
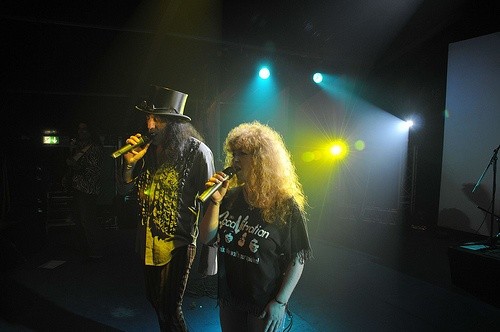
112,134,151,158
198,167,236,202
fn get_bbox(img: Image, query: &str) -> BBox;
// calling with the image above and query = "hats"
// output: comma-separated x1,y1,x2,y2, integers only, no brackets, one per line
134,85,191,122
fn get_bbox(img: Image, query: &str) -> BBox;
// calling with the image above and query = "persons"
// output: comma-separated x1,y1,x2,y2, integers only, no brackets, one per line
116,85,216,332
199,122,313,332
64,120,108,261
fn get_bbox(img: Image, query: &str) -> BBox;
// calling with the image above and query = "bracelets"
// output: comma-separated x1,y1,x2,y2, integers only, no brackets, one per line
124,162,138,170
272,297,293,332
